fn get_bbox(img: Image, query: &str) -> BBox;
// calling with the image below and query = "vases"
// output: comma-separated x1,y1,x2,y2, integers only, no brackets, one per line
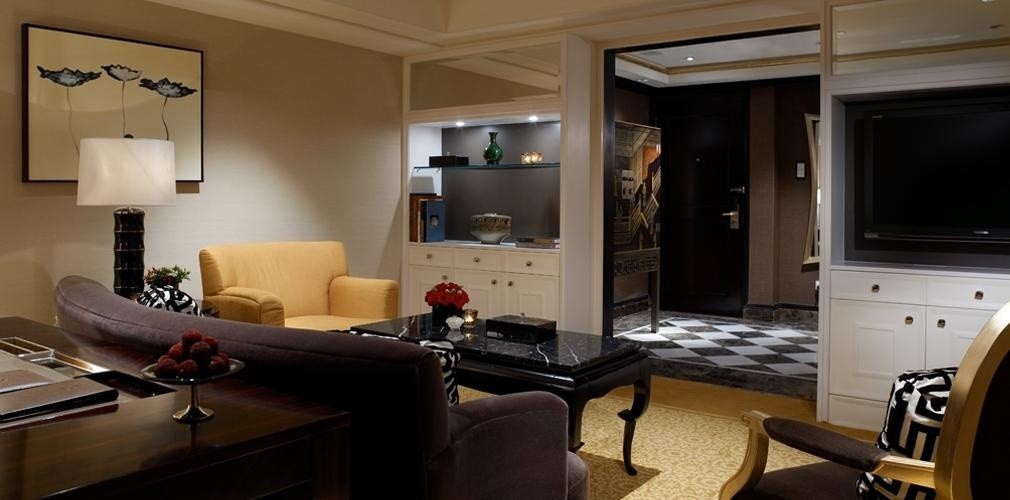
431,304,462,331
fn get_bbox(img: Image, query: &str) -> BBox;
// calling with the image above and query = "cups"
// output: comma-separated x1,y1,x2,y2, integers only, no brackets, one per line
531,152,543,164
520,152,531,165
463,308,478,330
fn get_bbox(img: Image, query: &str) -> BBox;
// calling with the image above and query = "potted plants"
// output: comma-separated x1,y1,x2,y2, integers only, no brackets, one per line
143,264,189,290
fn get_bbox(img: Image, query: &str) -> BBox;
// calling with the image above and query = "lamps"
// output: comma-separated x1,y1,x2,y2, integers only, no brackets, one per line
74,132,180,298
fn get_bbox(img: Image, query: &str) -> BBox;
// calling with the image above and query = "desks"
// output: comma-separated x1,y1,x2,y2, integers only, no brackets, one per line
190,295,220,318
0,313,352,499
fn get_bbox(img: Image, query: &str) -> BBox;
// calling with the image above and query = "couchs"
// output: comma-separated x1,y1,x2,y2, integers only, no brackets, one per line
199,239,400,332
55,268,593,500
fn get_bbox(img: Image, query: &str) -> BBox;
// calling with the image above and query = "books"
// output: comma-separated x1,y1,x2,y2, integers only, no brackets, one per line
410,193,447,243
516,236,560,249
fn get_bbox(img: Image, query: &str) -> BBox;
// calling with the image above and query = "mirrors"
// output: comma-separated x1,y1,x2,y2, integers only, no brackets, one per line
800,113,819,267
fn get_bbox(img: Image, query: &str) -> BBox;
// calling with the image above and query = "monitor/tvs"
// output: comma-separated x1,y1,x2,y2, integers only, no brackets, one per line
844,90,1009,257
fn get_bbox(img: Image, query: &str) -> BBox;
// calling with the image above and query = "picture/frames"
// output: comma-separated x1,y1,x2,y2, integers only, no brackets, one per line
18,21,206,190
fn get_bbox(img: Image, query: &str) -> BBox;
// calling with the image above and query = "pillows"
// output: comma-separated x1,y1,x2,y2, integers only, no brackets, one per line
135,288,198,317
854,366,960,500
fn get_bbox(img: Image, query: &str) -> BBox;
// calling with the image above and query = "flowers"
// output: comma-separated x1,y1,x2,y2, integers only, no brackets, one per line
424,280,470,308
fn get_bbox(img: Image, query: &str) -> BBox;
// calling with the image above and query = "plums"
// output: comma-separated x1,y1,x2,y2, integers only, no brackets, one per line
154,327,230,377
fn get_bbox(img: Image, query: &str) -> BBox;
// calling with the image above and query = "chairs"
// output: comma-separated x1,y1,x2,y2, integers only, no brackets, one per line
718,297,1010,500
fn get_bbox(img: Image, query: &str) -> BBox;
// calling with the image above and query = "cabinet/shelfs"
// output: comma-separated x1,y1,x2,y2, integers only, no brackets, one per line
454,247,592,334
411,247,453,315
815,270,1010,435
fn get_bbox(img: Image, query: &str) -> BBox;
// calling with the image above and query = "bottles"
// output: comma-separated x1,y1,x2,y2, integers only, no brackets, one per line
469,211,513,245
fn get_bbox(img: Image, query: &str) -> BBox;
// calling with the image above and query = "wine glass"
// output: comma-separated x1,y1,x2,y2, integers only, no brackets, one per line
142,356,245,424
